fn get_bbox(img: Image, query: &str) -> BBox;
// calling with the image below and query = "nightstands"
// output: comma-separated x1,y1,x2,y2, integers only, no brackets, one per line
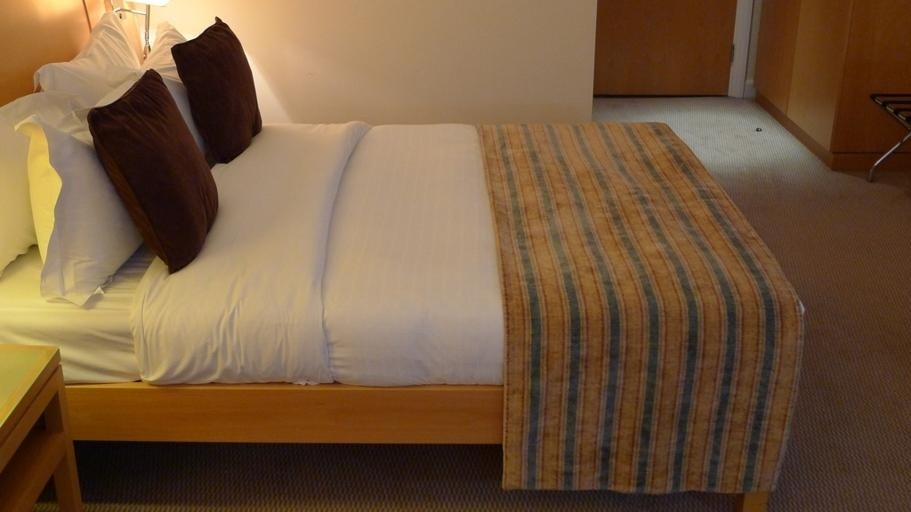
0,344,82,511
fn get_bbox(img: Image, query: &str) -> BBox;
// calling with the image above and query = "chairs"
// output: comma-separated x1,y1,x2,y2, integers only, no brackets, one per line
867,91,911,181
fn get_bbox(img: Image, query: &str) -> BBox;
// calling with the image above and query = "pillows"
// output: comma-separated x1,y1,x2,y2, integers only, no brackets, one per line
89,18,218,169
169,15,263,173
3,91,84,273
88,68,220,276
13,113,144,303
33,7,141,115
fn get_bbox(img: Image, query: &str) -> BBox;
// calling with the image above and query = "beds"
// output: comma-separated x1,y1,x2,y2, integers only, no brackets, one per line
0,2,807,510
